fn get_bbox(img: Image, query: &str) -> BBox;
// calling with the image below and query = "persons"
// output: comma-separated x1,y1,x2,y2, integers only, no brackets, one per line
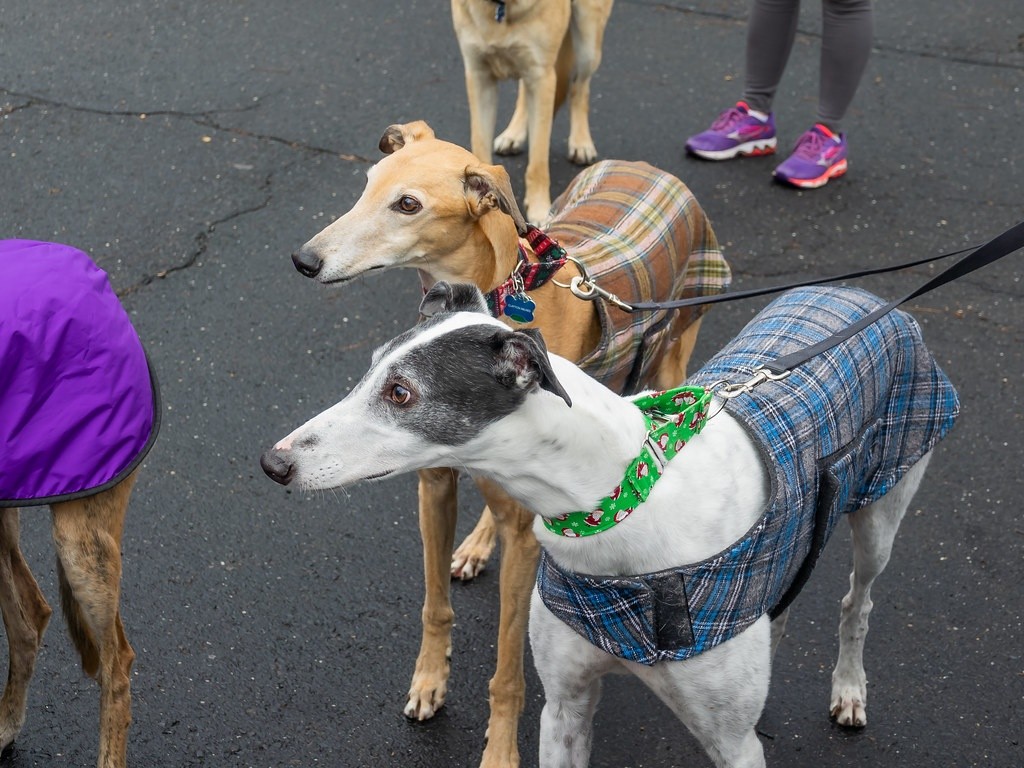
684,0,872,188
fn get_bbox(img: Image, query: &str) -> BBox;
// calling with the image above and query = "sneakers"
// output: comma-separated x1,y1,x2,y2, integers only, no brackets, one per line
770,126,848,188
684,100,778,160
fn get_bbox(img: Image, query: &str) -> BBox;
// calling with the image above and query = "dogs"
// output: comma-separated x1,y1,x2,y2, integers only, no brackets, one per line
260,281,959,767
299,118,733,768
441,1,614,231
1,235,158,766
